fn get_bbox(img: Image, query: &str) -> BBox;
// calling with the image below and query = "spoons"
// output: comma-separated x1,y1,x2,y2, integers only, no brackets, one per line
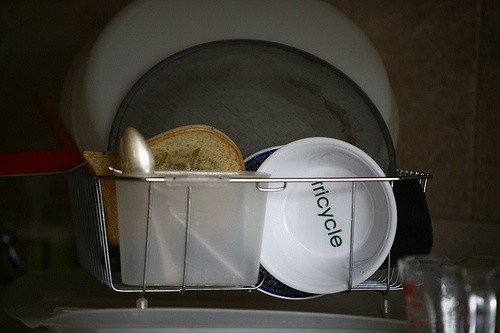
118,128,155,174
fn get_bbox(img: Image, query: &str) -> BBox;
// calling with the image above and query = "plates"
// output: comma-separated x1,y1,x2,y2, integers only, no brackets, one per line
256,137,398,294
243,144,325,300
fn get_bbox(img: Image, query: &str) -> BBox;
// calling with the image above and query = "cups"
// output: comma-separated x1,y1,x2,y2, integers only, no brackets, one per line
398,256,498,333
